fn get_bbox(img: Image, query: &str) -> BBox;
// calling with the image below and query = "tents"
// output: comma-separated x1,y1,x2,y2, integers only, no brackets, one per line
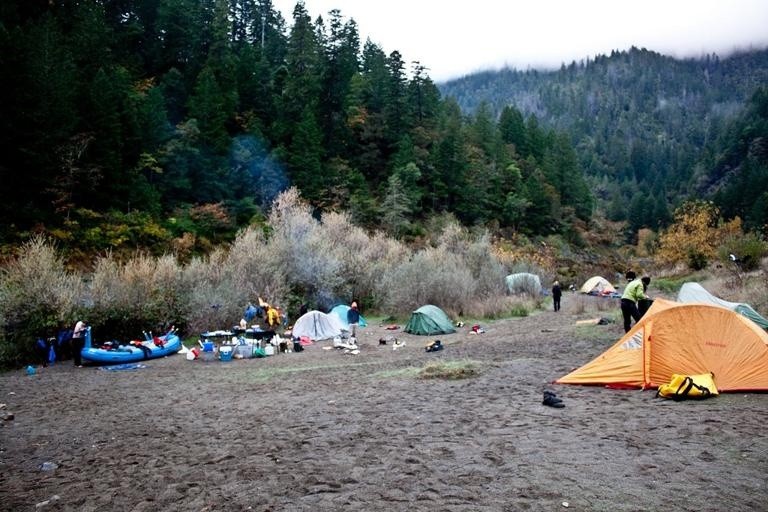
292,310,342,342
552,298,768,393
404,304,456,335
505,272,543,297
578,276,618,296
676,282,768,330
330,305,368,330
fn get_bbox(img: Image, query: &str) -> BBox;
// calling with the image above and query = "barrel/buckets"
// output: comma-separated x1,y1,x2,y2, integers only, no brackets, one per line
219,346,232,361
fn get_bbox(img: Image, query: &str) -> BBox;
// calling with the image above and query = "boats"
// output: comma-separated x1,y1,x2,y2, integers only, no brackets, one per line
80,326,181,363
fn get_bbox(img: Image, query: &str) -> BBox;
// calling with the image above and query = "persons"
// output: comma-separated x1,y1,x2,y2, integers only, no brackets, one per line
626,269,636,282
347,302,360,343
72,319,88,368
552,281,562,312
620,277,650,333
